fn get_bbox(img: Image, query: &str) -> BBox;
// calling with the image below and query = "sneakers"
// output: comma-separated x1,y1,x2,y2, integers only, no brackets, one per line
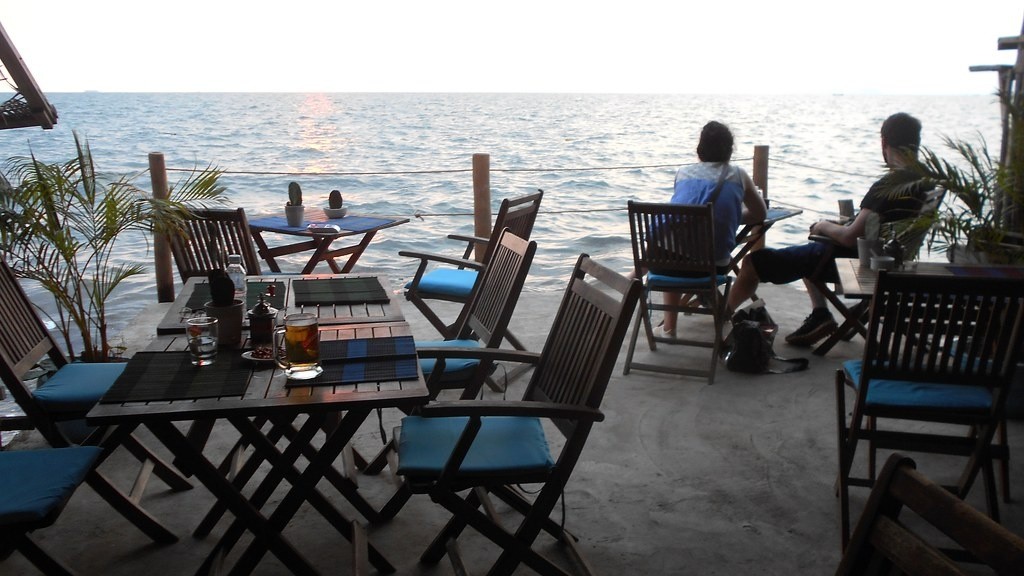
785,309,838,347
652,325,677,339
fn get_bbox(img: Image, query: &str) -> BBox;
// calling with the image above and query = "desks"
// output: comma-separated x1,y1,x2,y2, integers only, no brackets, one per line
834,257,1024,364
86,321,431,576
245,207,410,274
157,272,408,538
659,199,803,330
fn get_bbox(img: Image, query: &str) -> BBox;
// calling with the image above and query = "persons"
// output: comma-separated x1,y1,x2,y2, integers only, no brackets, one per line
724,113,944,345
626,119,767,339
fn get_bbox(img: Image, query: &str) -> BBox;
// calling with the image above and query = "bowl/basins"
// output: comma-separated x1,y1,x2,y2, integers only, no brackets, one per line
870,256,896,272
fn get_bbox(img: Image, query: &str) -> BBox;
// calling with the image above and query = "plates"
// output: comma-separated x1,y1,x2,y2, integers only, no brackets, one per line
241,350,275,361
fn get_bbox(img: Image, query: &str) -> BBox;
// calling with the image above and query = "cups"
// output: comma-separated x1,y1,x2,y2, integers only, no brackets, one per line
185,317,220,366
902,260,918,272
273,312,325,380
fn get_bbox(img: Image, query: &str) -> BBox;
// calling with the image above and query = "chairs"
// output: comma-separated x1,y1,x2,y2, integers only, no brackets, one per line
0,187,1024,576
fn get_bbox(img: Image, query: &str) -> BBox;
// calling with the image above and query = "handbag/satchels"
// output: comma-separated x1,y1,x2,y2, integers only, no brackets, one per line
718,321,808,375
650,208,712,272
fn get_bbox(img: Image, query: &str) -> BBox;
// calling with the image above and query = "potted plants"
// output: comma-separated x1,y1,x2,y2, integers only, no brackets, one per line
854,211,886,267
0,129,244,445
323,190,347,218
203,269,244,346
285,181,305,226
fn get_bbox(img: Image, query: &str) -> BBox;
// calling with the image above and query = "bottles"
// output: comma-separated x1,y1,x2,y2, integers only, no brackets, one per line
225,254,248,323
